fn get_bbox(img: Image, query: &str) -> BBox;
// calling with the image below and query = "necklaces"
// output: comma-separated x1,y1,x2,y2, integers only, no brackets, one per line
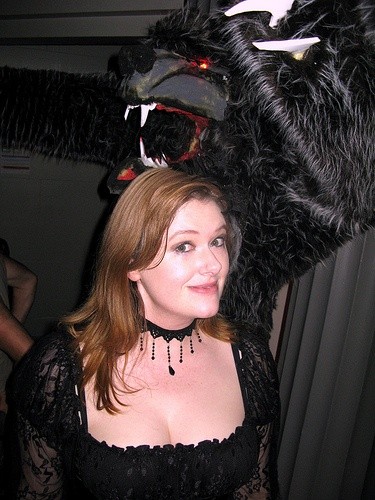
132,312,203,378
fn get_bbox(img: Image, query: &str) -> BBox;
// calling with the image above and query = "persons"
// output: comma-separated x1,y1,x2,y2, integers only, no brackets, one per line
0,254,38,431
0,168,287,500
